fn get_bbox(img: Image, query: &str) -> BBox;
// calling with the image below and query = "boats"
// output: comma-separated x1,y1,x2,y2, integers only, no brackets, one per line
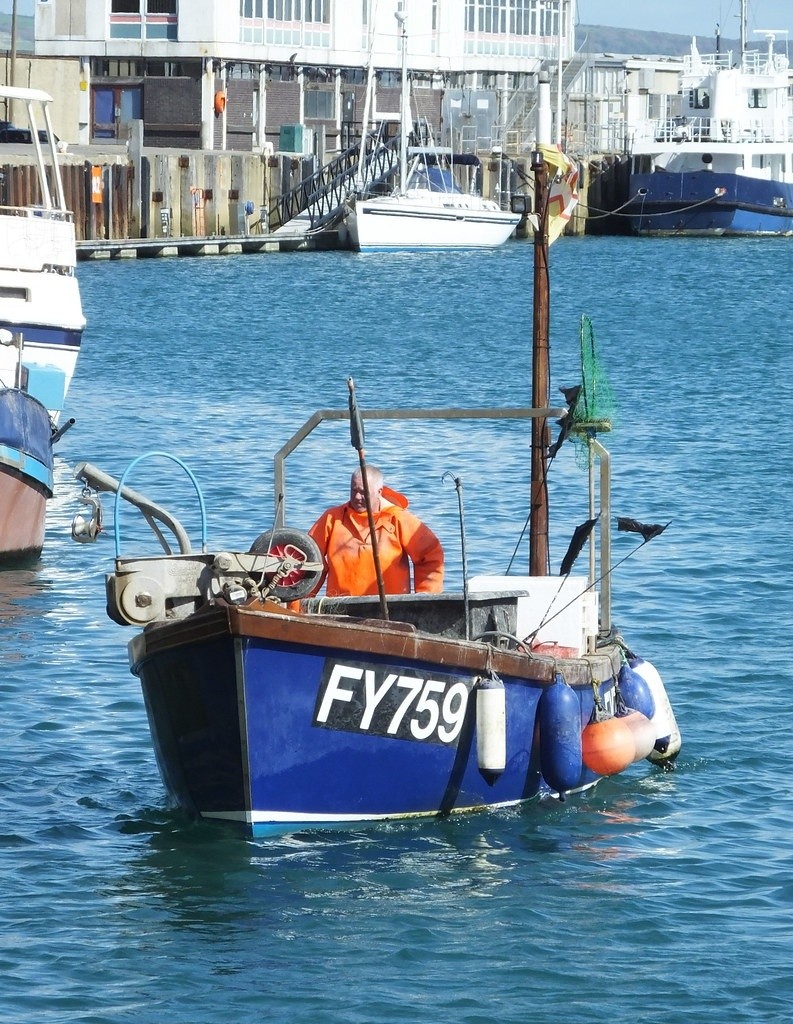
0,83,88,433
0,334,76,572
599,0,793,241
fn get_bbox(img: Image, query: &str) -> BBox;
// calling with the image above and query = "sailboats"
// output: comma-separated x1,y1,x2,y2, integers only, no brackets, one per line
70,150,684,842
341,9,524,254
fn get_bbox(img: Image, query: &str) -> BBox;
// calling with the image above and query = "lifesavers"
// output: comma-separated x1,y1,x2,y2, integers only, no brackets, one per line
247,528,323,600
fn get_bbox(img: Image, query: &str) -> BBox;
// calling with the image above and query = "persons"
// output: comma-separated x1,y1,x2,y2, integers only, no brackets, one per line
307,465,444,598
